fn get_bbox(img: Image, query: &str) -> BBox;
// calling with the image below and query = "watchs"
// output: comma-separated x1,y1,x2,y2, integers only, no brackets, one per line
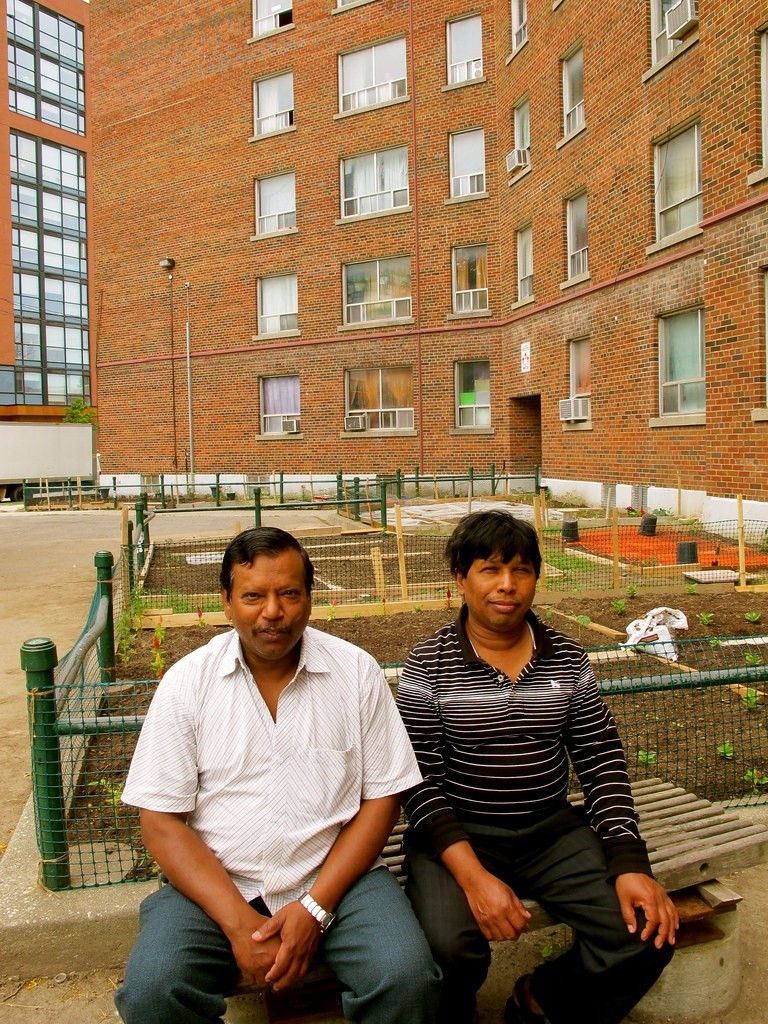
298,892,337,933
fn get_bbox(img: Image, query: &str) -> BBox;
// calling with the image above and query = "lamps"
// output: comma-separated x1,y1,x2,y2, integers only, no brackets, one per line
160,258,174,268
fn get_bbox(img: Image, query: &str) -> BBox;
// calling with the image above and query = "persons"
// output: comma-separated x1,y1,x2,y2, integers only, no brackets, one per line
114,525,444,1024
396,509,679,1024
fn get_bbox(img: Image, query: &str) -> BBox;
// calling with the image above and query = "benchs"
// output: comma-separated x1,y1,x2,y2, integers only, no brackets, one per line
223,777,767,1024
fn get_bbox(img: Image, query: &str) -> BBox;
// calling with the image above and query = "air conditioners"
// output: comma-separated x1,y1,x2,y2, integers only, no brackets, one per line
471,59,483,79
344,416,365,429
558,398,588,419
664,0,699,41
505,149,527,172
281,419,300,432
